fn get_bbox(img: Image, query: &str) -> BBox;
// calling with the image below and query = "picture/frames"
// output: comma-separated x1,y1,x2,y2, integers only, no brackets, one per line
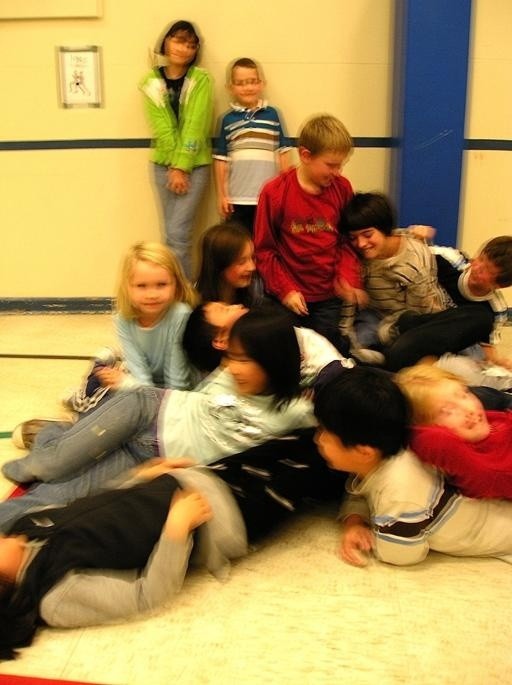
57,45,104,109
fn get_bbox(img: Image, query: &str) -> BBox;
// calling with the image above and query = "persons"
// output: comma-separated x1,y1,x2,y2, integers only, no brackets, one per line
1,20,511,660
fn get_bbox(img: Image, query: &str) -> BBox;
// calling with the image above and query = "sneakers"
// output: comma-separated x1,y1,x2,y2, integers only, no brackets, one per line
10,416,75,452
